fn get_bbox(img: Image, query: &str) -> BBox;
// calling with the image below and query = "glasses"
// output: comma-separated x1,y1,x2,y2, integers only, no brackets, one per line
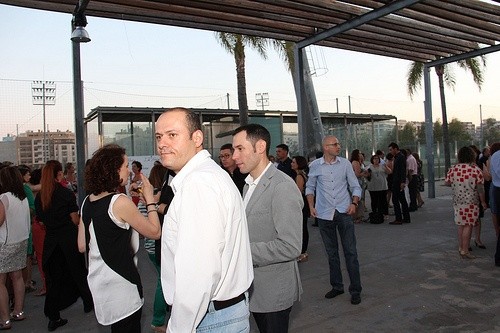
218,153,232,159
326,142,341,147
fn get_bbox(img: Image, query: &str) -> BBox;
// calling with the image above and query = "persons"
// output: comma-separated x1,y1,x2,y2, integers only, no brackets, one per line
218,144,248,196
78,143,161,333
350,143,425,225
0,160,93,333
232,124,305,333
154,108,254,333
445,141,500,266
306,136,363,305
130,161,174,333
270,144,324,262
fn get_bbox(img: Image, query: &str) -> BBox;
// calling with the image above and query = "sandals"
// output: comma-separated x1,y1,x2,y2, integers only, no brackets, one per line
9,310,25,321
0,320,11,330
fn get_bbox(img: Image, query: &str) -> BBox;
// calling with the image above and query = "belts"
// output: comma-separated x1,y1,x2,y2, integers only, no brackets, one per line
166,291,246,312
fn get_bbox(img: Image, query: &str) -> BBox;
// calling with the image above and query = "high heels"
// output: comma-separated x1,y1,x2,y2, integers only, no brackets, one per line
474,240,486,250
151,324,166,333
296,253,309,263
458,248,478,260
418,201,425,207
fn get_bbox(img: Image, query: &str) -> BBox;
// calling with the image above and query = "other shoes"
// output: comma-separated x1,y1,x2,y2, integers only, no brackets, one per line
408,207,418,212
48,317,68,331
389,220,402,224
351,293,362,304
36,290,47,296
325,289,344,299
402,219,410,223
84,303,94,313
